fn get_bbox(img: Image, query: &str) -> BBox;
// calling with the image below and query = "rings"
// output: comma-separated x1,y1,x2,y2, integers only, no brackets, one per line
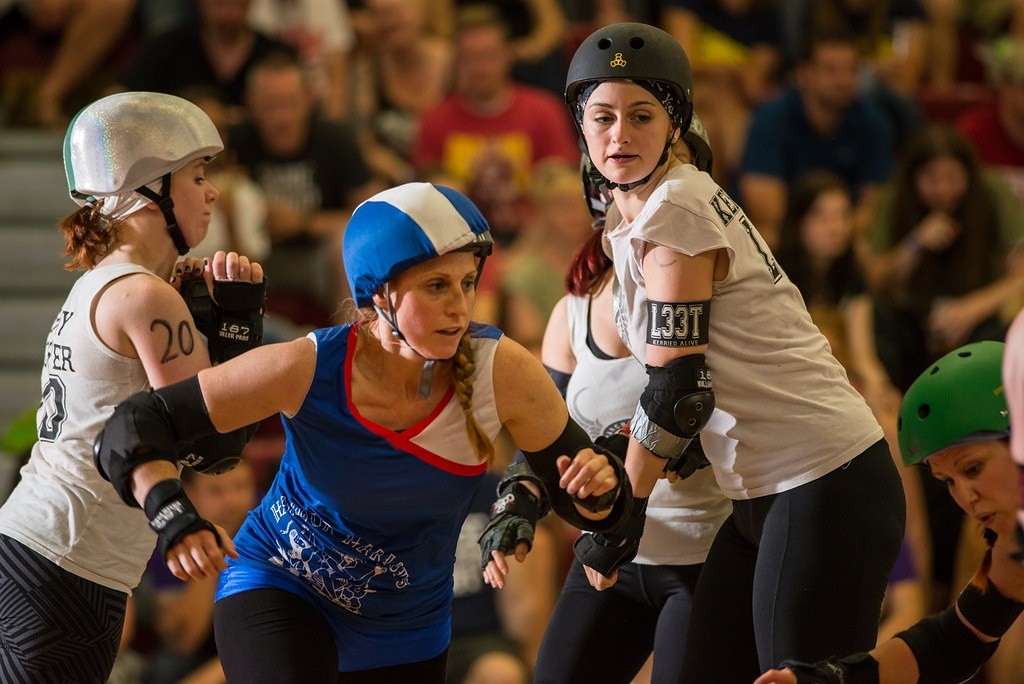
227,275,240,279
239,279,251,283
214,276,226,279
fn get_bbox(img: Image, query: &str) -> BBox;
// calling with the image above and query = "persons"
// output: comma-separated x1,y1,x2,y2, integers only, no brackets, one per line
556,18,907,684
0,93,267,684
475,105,761,684
751,340,1024,684
89,178,622,683
28,0,1024,682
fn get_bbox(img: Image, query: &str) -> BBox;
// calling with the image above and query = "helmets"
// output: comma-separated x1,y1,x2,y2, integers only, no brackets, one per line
563,22,695,133
581,112,713,182
342,182,495,307
896,341,1010,467
62,91,223,208
